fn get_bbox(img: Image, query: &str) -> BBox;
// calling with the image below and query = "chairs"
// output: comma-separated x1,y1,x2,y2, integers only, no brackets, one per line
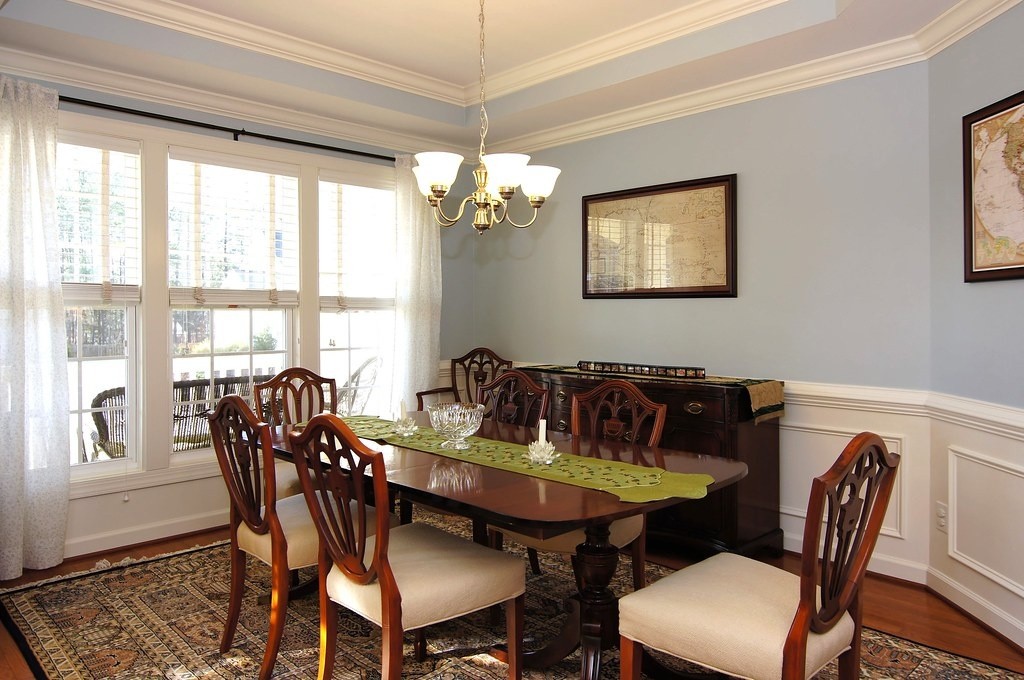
416,347,513,412
207,393,401,680
254,367,337,426
617,429,902,680
478,370,550,429
321,353,384,415
487,380,666,595
289,413,527,680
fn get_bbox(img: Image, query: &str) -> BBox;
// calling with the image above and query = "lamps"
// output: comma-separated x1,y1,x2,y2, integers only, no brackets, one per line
411,0,561,237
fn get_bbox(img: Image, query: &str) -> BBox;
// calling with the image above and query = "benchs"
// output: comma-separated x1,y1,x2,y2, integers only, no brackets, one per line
88,374,284,462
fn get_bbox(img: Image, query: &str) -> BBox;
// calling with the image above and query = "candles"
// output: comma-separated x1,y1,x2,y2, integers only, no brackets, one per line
538,419,547,447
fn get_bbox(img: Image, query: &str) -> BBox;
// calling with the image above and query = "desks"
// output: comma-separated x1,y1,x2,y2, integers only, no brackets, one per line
240,410,749,680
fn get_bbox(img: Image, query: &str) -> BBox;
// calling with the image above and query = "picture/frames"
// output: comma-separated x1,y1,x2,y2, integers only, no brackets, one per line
580,172,739,302
961,88,1024,284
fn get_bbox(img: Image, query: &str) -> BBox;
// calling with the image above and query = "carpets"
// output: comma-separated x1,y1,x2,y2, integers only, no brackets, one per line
0,491,1024,680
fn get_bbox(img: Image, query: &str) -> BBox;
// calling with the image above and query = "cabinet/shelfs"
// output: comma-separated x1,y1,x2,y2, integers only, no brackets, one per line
506,364,786,566
